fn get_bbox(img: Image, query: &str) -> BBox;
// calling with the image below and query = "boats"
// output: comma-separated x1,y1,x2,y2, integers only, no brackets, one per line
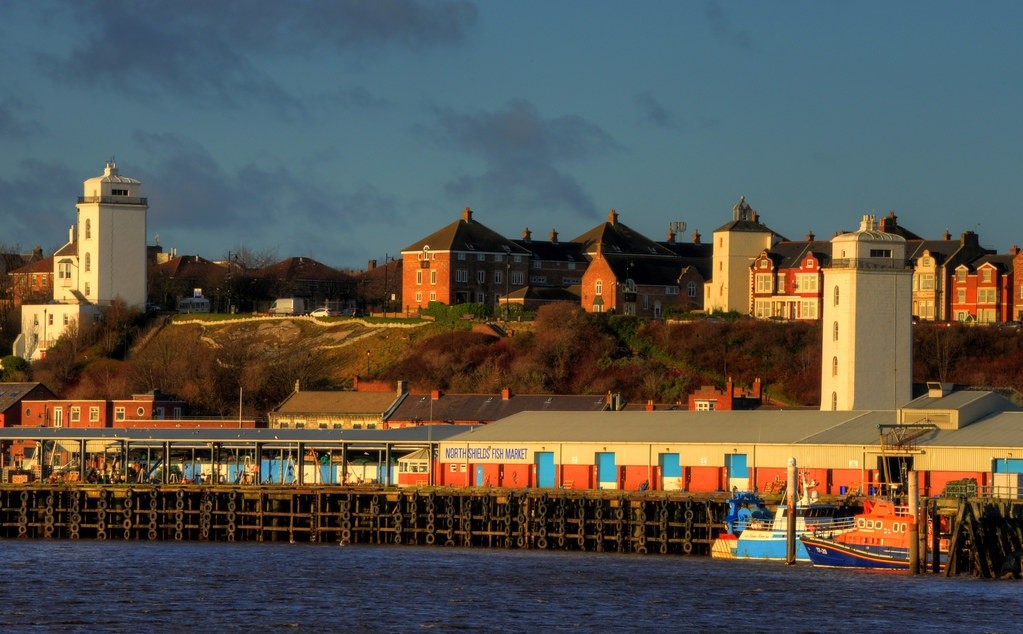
711,458,972,574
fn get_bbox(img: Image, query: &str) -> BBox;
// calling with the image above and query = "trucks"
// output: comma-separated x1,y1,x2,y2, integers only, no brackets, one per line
268,298,304,316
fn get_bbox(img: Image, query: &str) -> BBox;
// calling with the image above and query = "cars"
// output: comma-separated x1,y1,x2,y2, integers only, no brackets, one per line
310,308,335,318
502,243,511,322
997,320,1022,332
933,320,962,327
340,308,364,319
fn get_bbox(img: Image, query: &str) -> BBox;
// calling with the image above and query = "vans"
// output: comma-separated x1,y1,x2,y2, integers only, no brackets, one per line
177,298,210,315
